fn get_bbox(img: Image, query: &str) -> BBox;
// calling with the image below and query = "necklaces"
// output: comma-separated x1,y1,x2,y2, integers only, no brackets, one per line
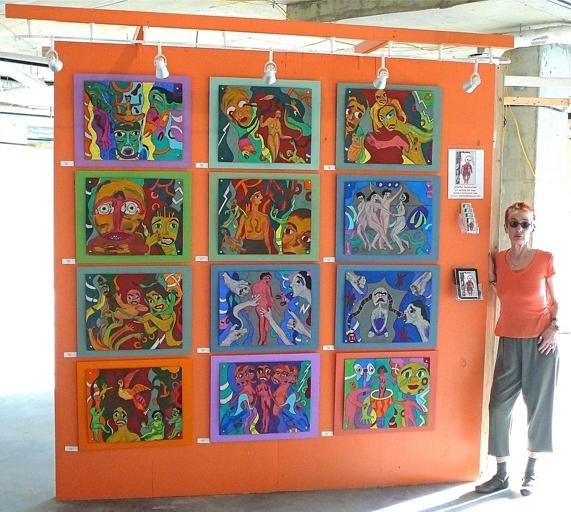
509,254,520,268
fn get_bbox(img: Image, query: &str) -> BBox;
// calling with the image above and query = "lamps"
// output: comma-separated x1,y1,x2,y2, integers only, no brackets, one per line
45,36,481,94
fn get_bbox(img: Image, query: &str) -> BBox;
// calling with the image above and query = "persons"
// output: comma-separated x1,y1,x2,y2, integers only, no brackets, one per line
472,200,560,497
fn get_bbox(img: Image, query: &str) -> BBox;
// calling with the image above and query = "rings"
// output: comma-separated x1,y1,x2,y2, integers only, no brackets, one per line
539,335,544,339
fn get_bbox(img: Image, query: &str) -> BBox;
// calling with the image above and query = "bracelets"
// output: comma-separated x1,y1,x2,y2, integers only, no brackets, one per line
490,280,497,288
551,323,559,331
552,317,559,320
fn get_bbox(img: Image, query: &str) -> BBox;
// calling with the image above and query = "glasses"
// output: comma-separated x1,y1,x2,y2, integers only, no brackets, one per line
508,220,532,228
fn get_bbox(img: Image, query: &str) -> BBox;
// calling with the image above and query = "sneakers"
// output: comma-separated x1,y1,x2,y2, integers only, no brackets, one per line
521,474,536,497
475,473,510,495
